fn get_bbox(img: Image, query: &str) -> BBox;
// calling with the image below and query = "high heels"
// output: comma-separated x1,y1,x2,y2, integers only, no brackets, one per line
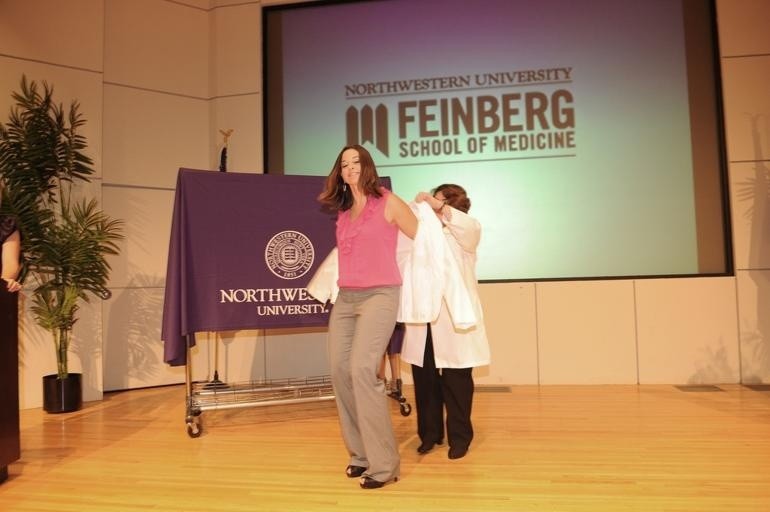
448,444,468,459
346,464,398,489
417,438,443,453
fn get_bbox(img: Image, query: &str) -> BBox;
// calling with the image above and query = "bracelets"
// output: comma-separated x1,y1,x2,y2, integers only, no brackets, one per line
438,202,447,211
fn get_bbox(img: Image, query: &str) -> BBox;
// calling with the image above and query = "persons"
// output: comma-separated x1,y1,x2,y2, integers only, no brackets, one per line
316,145,420,491
376,322,406,397
1,187,24,295
398,184,488,460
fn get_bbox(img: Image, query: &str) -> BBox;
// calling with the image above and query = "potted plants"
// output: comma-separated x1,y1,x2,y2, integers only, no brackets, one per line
0,73,124,413
